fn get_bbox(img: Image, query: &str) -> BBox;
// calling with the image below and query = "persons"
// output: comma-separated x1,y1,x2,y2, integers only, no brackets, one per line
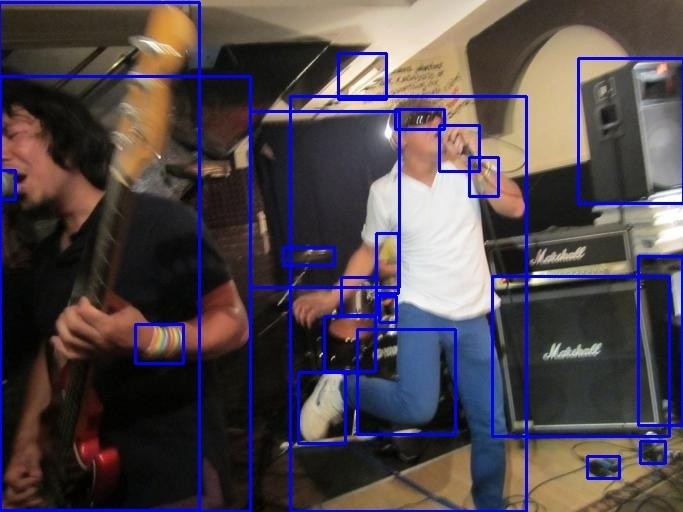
290,97,526,512
0,77,250,512
377,233,399,293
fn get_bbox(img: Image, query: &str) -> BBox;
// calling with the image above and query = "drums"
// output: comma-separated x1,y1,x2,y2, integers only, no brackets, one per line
324,316,376,372
358,329,455,434
341,278,376,315
379,297,397,323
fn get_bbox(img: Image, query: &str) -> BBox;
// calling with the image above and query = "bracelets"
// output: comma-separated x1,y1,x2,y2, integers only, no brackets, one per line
470,157,498,197
136,325,183,364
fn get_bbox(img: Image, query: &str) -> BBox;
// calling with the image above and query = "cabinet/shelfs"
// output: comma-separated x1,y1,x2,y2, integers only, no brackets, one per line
639,256,683,426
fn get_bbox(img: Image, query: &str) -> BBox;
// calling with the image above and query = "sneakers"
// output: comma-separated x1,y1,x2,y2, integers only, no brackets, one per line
298,372,345,443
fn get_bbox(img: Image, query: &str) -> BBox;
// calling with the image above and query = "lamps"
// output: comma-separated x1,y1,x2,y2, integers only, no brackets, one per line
338,54,387,99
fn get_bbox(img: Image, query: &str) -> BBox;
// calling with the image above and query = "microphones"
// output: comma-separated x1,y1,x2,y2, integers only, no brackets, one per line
439,125,479,172
0,170,16,199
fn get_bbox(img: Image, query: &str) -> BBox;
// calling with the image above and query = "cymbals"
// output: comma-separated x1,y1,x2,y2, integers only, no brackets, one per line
284,247,335,265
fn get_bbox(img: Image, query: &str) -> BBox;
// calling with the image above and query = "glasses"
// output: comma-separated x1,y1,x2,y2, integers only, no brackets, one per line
395,110,445,130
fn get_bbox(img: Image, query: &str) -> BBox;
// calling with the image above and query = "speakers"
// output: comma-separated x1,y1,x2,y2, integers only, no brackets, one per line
579,59,683,203
250,111,399,288
493,277,670,437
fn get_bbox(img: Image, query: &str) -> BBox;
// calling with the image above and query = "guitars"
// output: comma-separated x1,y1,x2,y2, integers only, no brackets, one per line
0,2,199,510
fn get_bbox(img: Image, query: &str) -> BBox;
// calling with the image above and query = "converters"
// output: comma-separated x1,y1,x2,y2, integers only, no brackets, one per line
640,441,665,463
588,457,619,478
